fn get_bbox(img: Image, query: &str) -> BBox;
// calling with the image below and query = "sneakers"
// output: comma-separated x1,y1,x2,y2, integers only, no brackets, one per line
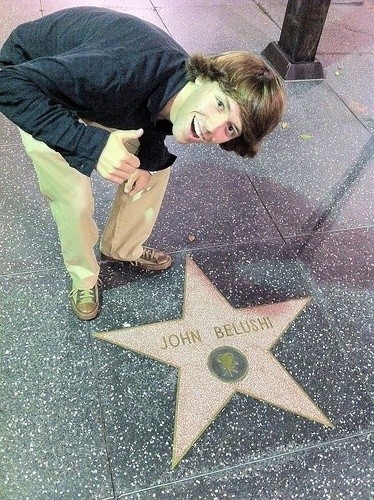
70,277,103,320
100,245,171,270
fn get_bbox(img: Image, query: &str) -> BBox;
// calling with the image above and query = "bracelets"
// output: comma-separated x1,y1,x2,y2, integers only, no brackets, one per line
148,170,158,175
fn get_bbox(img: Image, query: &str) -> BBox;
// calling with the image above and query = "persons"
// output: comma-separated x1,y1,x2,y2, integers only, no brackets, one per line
0,6,285,319
